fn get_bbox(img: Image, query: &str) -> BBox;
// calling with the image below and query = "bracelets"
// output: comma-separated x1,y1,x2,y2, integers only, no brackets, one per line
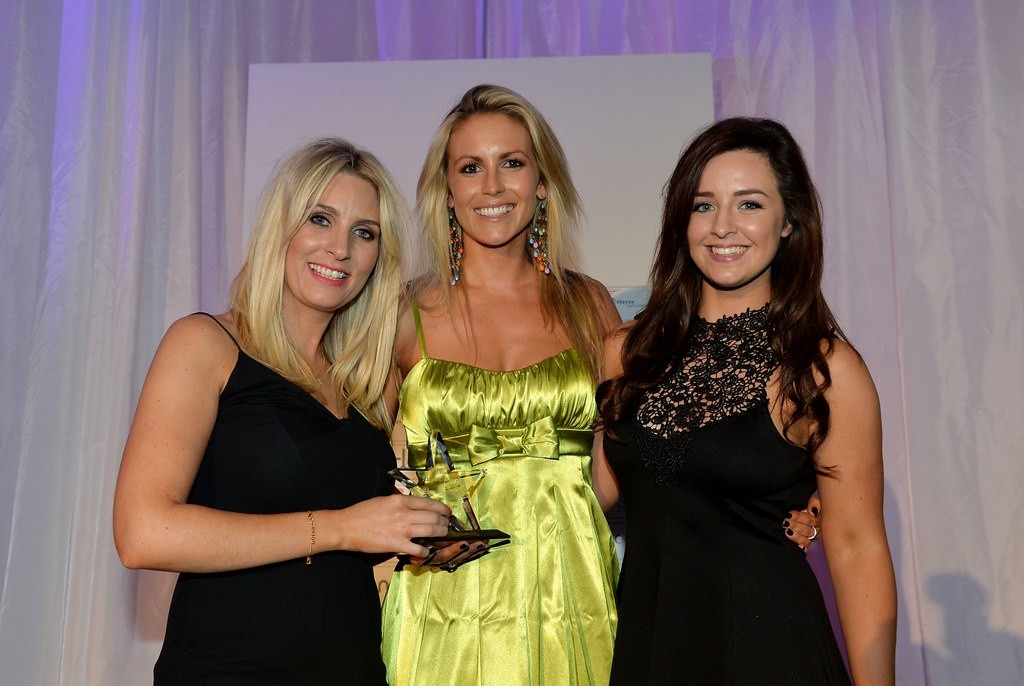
307,510,316,565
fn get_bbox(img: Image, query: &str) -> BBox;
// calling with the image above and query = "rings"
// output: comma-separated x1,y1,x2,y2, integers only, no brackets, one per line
808,526,817,539
447,562,456,568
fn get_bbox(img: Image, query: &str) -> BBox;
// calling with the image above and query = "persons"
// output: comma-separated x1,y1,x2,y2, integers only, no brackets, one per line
112,139,487,686
368,85,823,686
594,117,898,686
